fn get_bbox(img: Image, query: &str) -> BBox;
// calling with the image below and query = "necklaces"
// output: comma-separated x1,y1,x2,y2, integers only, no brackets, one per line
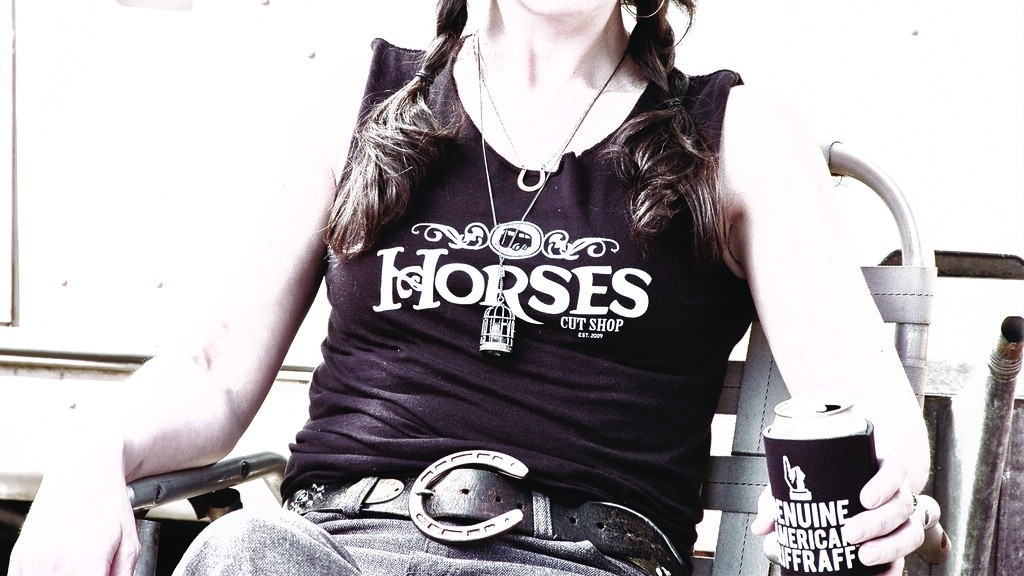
472,32,631,357
472,29,631,192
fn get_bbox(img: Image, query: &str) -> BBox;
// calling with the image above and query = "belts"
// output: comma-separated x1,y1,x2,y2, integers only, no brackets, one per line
282,448,692,576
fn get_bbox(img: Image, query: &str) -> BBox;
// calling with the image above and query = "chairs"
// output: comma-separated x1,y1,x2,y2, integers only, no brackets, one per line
126,135,938,576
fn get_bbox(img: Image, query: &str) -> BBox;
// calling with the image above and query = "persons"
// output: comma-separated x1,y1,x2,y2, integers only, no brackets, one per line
7,1,930,576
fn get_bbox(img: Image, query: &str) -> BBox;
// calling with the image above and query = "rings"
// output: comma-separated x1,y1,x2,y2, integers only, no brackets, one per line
911,491,918,515
